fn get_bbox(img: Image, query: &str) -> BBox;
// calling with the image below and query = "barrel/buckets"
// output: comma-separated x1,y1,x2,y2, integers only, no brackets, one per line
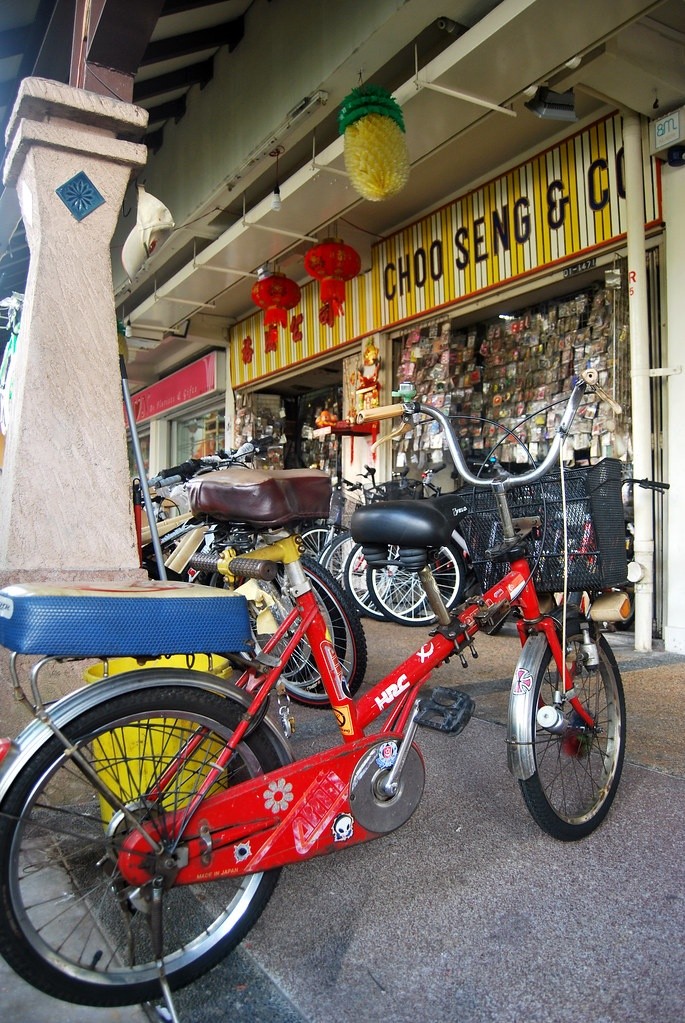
82,653,235,845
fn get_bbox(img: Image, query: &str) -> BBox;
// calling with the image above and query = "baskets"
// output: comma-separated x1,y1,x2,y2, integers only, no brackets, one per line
325,490,363,530
363,478,424,504
457,457,628,595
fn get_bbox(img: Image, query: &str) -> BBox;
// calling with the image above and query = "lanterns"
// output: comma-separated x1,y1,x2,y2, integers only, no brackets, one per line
303,236,361,316
251,271,301,328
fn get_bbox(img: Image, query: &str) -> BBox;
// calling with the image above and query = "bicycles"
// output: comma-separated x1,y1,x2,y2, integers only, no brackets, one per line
139,435,511,708
0,367,637,1023
